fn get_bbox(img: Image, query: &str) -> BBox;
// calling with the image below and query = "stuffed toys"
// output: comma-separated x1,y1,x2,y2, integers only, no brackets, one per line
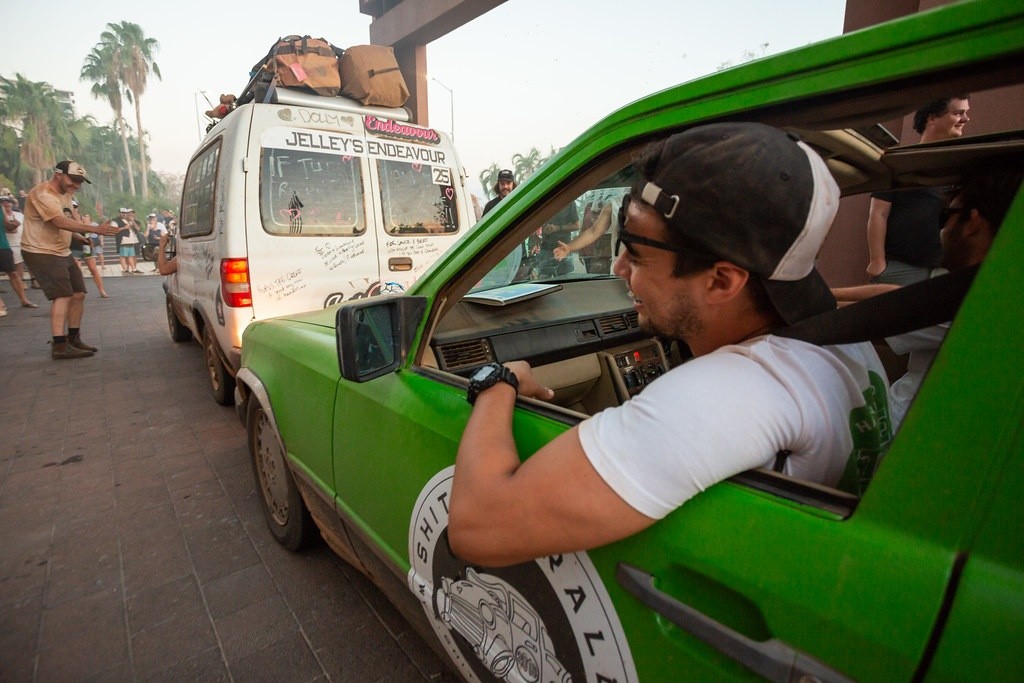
206,94,236,118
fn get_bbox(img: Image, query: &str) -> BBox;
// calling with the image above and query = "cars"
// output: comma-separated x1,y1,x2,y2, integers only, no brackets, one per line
231,0,1024,683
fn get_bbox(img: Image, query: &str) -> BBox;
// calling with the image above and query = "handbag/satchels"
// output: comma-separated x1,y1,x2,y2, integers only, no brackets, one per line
249,35,341,96
339,45,411,108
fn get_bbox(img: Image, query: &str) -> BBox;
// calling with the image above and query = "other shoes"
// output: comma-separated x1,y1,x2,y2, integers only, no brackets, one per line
101,292,111,297
101,266,106,269
0,286,7,293
47,338,94,359
21,281,28,290
68,333,98,352
122,270,133,275
22,302,38,308
0,306,7,316
132,270,144,274
31,280,42,289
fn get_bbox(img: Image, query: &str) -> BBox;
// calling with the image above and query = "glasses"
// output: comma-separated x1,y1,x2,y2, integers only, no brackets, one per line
937,207,967,229
6,193,12,196
612,193,719,259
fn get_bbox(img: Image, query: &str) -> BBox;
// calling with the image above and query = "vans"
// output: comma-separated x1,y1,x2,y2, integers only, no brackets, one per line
139,88,477,408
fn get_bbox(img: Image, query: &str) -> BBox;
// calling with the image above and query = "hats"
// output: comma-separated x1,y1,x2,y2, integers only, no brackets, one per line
118,207,127,213
0,196,12,203
127,209,134,213
55,160,91,184
497,170,514,182
638,122,842,327
149,213,158,218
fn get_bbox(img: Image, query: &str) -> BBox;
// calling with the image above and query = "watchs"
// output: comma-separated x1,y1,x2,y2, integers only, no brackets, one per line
466,364,519,407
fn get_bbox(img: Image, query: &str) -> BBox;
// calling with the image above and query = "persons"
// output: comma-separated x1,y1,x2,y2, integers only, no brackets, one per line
72,201,110,298
448,124,890,568
85,214,108,270
16,189,28,212
0,200,39,316
866,92,970,287
481,170,517,217
147,214,167,271
0,196,27,290
829,184,1010,438
21,161,119,358
538,199,581,281
126,208,142,262
163,210,178,235
553,191,628,277
159,233,177,276
0,188,19,210
109,208,144,274
